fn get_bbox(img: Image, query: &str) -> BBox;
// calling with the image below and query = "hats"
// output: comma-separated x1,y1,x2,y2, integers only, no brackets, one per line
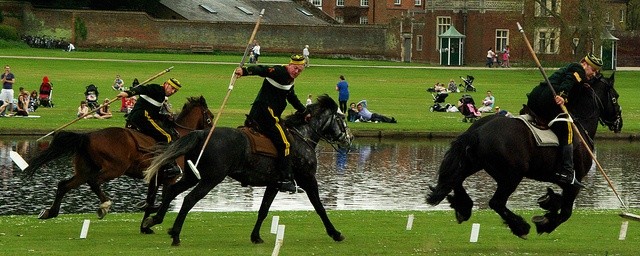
289,53,305,65
585,52,604,70
167,77,182,90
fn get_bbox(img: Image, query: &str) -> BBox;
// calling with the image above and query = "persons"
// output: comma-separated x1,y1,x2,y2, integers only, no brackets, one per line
0,65,15,115
117,77,182,172
356,100,397,123
447,79,458,93
77,101,93,119
94,97,112,120
477,91,495,113
130,79,140,90
235,54,311,193
28,90,39,111
487,48,493,67
111,74,124,90
306,94,312,106
347,102,358,121
302,45,309,68
503,51,508,67
527,53,603,188
120,97,137,115
501,46,510,68
0,100,8,117
495,107,514,118
250,40,261,64
483,97,491,106
18,86,30,100
13,95,28,116
23,93,29,107
335,75,350,113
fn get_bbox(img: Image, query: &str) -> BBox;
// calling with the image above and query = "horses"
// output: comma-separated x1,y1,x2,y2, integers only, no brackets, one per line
425,73,624,239
12,94,214,233
141,95,355,247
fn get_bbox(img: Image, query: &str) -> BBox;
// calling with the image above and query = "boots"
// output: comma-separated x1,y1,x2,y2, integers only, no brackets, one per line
278,154,304,193
558,142,586,189
